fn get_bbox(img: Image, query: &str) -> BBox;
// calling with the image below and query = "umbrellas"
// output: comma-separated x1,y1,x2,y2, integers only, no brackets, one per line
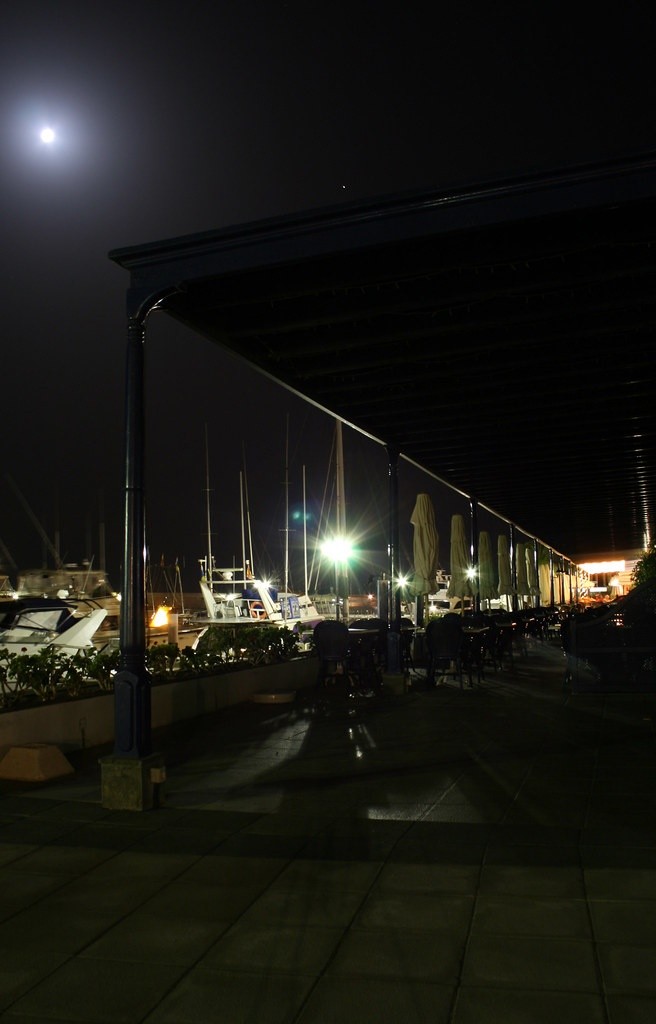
410,494,439,627
447,515,478,617
517,544,531,609
525,548,541,608
498,536,518,612
480,532,500,614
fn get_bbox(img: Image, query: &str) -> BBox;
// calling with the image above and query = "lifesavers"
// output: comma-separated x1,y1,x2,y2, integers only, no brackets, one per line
250,601,266,619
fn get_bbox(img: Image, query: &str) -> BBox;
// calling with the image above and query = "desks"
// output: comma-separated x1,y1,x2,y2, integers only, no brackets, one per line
497,622,517,660
411,626,490,685
399,624,419,674
310,628,379,682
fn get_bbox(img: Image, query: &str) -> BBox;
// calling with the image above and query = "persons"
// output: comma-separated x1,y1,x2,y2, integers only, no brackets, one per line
595,594,610,607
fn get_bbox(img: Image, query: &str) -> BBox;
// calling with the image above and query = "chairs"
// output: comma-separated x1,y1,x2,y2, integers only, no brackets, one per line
313,602,587,692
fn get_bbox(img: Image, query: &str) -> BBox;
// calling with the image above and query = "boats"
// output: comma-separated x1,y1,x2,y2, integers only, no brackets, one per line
0,413,336,693
423,570,473,616
